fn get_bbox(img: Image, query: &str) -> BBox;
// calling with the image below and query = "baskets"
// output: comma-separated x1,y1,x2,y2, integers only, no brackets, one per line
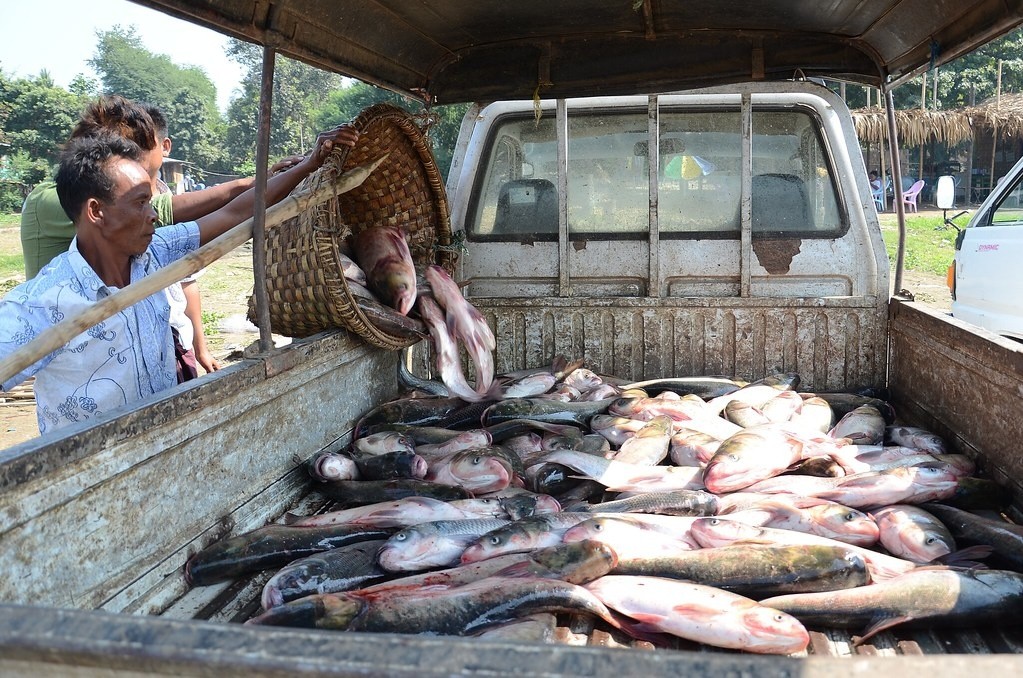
245,106,454,352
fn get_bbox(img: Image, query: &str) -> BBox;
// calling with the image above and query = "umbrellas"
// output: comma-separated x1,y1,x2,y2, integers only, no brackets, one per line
667,150,716,180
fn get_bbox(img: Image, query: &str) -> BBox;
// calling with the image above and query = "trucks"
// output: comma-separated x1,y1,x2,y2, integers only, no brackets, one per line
938,154,1023,344
0,0,1023,678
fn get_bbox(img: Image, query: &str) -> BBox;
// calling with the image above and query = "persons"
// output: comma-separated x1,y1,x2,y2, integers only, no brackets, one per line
20,99,306,282
869,171,881,199
0,128,360,436
140,103,220,383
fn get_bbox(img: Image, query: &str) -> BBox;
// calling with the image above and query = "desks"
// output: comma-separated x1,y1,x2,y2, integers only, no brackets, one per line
959,186,994,208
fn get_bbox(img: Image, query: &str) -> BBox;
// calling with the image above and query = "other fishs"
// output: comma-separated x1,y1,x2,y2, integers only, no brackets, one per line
184,225,1023,655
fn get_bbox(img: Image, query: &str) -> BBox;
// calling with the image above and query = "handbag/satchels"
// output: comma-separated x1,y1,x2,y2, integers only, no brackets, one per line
168,326,198,384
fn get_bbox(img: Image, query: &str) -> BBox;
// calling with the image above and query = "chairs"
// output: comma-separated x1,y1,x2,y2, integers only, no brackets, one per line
870,177,925,214
997,177,1021,207
733,173,818,232
491,180,559,235
